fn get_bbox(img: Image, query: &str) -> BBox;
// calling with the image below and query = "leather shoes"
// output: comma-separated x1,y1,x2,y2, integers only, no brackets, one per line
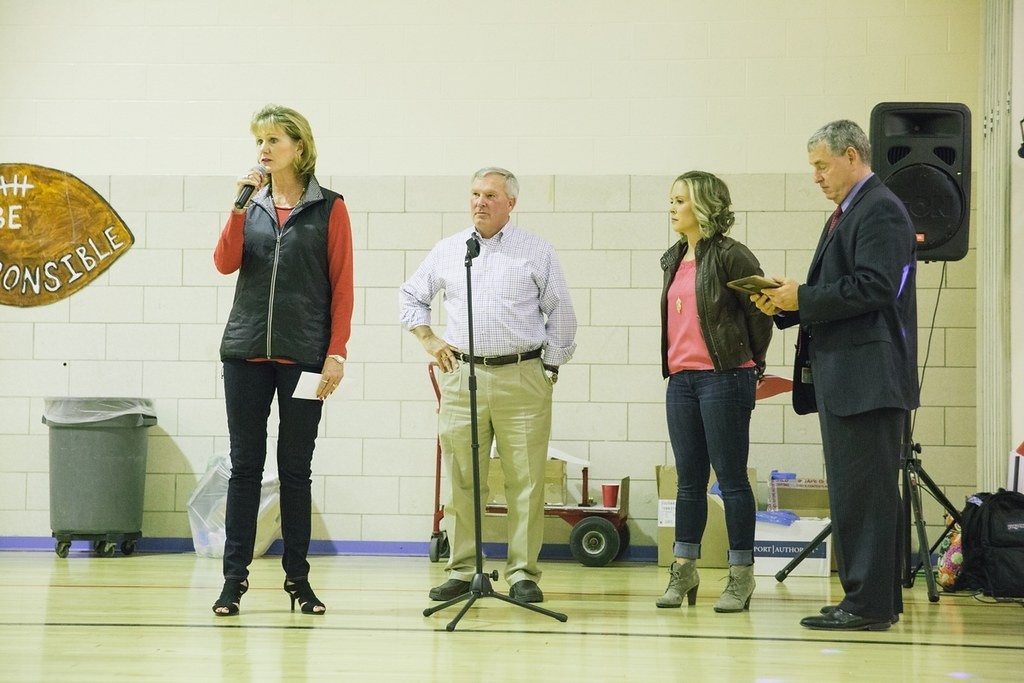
820,605,899,623
801,614,891,630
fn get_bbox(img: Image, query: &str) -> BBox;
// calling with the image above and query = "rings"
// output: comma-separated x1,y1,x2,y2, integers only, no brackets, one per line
332,383,337,386
320,379,328,383
443,358,448,361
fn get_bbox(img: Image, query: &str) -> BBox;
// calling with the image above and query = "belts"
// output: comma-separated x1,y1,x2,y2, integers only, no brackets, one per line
453,347,541,367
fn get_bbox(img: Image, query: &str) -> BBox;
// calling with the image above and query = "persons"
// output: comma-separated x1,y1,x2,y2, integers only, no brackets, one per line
399,166,576,602
657,170,773,612
749,118,918,632
211,105,354,615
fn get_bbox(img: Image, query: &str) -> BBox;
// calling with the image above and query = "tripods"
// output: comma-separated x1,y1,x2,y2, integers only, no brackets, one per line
423,239,568,633
775,405,964,604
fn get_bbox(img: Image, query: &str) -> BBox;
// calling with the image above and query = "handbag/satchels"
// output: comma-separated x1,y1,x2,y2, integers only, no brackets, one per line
937,513,962,590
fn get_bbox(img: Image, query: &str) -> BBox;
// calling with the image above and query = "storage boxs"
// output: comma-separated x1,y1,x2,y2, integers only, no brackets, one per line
752,517,831,577
768,478,838,571
655,464,757,568
488,456,567,505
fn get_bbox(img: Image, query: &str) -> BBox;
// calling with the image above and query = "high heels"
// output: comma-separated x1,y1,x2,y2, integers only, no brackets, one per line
284,578,326,615
212,580,249,615
713,565,755,612
656,559,700,608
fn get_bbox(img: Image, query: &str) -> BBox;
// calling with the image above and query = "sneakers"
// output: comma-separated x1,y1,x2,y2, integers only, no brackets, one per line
509,580,543,602
429,580,469,601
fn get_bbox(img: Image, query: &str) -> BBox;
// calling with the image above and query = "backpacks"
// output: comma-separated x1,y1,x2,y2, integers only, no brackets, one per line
962,489,1024,597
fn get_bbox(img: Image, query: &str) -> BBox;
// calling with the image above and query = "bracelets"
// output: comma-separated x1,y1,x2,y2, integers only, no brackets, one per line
326,354,345,363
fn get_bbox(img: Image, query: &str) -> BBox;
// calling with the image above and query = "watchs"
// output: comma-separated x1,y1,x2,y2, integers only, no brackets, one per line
545,369,558,384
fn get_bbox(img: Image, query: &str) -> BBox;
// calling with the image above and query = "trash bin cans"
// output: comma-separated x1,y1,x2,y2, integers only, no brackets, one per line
41,395,158,558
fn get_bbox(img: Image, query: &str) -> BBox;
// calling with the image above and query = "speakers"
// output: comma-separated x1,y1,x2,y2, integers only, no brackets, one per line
869,102,972,264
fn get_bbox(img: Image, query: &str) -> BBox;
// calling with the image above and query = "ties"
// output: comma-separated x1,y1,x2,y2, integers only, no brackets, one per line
828,205,842,233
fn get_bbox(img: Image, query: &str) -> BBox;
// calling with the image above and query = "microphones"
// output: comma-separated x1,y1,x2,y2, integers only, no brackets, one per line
234,164,266,209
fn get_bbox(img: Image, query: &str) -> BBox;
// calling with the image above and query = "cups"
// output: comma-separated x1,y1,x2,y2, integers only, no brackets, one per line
602,485,619,508
